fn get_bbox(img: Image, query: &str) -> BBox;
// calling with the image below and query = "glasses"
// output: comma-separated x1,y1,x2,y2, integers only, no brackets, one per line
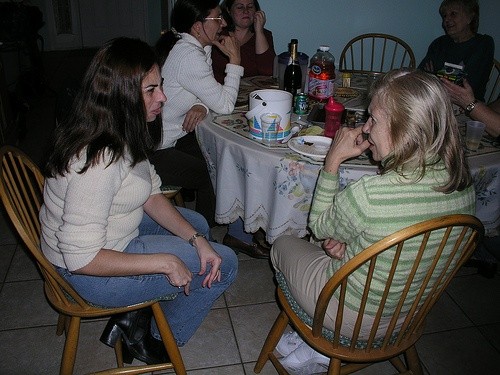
205,12,224,22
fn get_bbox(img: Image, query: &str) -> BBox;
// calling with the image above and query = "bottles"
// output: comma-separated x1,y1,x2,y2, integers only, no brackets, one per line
283,38,302,107
308,45,336,112
324,96,345,139
342,73,351,88
366,71,384,94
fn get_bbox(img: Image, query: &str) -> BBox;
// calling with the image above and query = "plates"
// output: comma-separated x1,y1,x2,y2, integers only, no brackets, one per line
287,135,333,161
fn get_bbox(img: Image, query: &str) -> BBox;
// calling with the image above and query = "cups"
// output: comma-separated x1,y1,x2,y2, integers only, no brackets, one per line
466,120,485,151
278,51,309,97
260,112,282,148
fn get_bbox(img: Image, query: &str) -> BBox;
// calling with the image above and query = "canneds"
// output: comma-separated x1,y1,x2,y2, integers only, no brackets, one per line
293,93,309,115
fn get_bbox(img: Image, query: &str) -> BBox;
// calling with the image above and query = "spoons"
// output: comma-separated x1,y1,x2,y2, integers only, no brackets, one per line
281,126,299,143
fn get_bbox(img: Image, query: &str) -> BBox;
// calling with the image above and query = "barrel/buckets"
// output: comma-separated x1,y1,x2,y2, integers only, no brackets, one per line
244,89,292,140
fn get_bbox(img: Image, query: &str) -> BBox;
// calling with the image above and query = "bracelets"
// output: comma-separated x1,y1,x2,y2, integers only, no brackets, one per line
189,233,207,247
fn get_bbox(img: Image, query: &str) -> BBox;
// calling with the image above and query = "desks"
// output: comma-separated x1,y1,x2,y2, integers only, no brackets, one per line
195,72,500,242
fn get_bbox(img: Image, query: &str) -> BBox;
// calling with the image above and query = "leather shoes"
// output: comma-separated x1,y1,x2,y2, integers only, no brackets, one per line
222,233,271,259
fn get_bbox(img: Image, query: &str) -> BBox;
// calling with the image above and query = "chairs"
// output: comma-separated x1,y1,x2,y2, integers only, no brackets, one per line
339,33,416,76
484,58,500,105
253,213,485,375
0,143,188,375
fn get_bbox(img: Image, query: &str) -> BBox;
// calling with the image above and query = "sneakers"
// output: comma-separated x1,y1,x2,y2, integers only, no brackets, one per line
276,331,347,375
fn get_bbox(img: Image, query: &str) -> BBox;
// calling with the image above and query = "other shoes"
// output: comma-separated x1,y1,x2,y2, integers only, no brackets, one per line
204,208,229,227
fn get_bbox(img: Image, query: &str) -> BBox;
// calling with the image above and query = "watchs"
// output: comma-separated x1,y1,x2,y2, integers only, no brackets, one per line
466,99,479,113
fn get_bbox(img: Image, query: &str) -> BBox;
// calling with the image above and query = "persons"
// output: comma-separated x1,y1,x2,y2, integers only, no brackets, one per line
211,0,276,85
270,67,475,375
418,0,495,100
39,38,238,366
441,76,500,140
148,0,270,260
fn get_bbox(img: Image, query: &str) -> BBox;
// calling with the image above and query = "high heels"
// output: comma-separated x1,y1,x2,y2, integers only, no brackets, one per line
98,307,172,365
